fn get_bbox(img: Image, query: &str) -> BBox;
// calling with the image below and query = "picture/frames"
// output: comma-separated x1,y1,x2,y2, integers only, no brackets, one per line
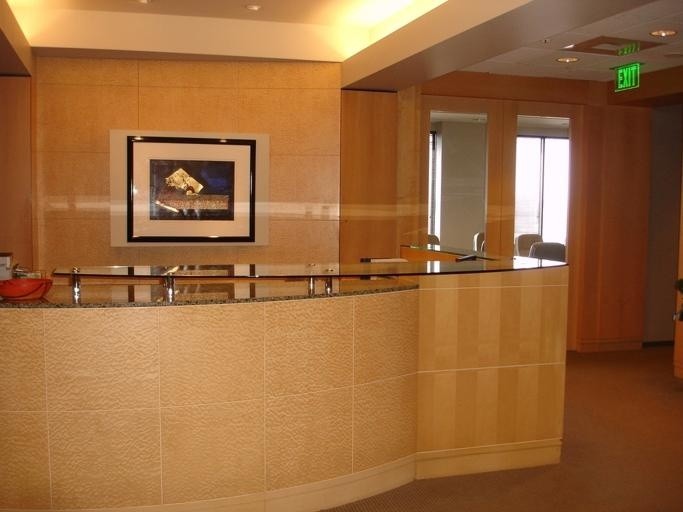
127,136,256,243
128,261,256,300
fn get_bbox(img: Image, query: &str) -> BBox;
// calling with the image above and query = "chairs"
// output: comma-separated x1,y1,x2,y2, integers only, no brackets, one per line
473,232,565,262
428,234,440,244
